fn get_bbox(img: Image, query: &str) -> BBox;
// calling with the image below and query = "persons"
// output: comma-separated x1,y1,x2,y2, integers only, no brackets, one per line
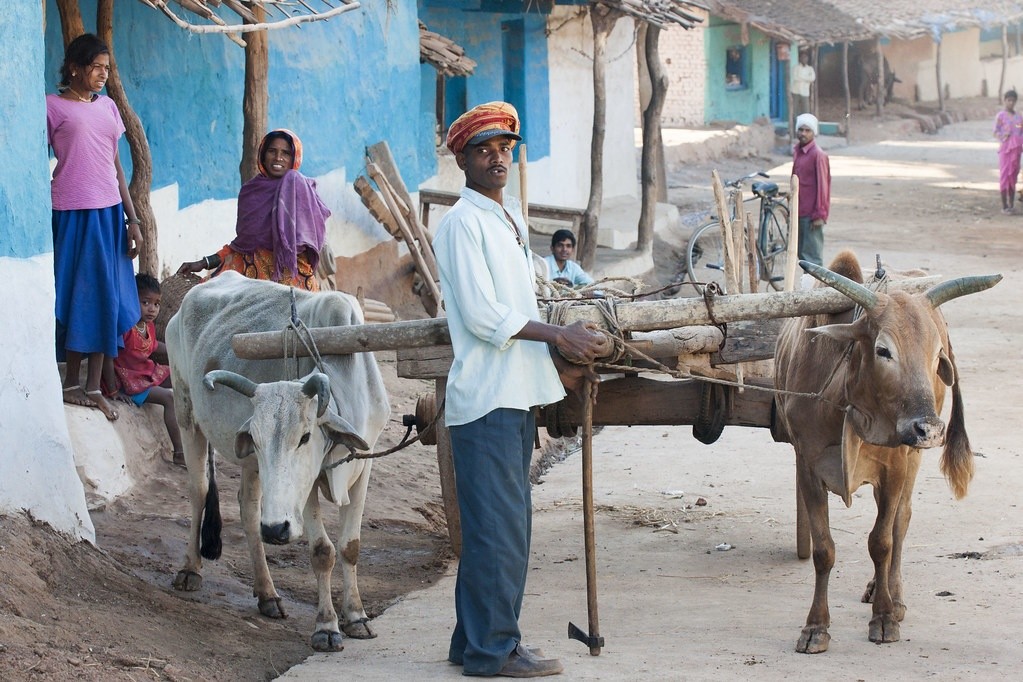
102,271,188,467
175,128,332,292
791,51,816,121
993,89,1023,226
791,112,831,288
544,228,604,299
46,33,145,420
432,102,606,678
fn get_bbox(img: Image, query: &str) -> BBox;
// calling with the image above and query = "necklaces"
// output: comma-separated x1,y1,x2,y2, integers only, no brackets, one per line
59,84,94,102
135,322,147,333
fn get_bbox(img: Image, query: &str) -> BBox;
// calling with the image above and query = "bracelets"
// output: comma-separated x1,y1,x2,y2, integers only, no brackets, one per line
125,219,142,225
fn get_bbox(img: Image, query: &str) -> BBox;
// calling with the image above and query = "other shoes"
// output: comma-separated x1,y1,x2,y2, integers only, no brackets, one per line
501,642,562,677
1001,206,1014,215
521,645,545,656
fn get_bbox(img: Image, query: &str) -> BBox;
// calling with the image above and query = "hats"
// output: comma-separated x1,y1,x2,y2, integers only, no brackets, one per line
467,128,521,145
445,100,521,156
796,113,818,137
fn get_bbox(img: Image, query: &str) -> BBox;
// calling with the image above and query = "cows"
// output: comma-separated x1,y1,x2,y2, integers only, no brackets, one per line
161,270,393,652
770,249,1003,655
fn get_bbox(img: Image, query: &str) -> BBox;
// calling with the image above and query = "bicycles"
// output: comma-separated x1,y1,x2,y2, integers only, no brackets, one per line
686,173,792,295
